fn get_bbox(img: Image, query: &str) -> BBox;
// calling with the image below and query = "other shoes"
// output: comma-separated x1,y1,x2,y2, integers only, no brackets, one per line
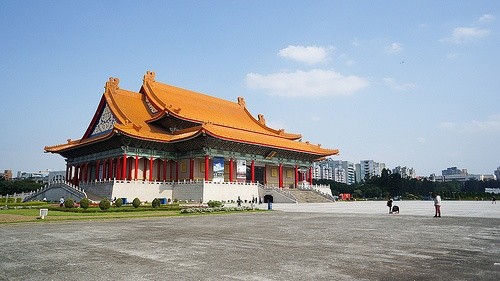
389,212,398,214
434,214,441,217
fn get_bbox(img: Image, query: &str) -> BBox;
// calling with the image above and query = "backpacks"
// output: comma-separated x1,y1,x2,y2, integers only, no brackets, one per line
387,200,391,206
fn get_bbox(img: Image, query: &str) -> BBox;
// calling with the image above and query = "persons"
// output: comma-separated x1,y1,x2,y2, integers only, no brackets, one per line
113,197,116,201
59,197,65,206
492,196,496,204
236,196,243,207
433,193,441,218
386,198,393,213
392,205,399,214
